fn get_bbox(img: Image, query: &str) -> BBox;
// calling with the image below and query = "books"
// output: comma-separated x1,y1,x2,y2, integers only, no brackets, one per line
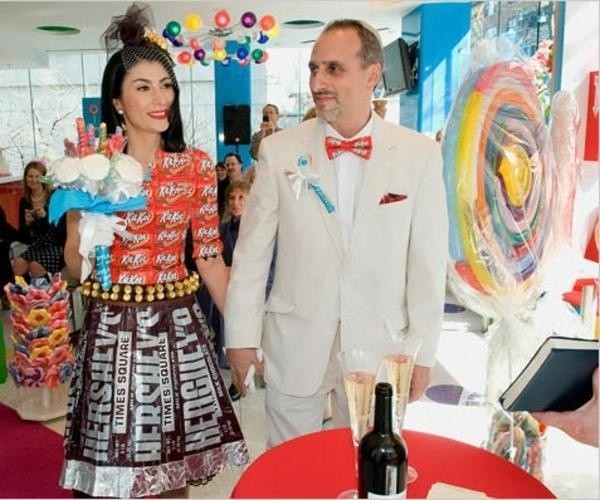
497,333,600,413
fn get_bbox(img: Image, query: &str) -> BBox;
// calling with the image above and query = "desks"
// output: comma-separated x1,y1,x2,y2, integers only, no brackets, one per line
0,183,24,230
230,427,560,499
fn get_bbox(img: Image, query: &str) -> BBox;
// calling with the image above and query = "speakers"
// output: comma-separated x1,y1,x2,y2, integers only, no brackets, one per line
223,105,250,145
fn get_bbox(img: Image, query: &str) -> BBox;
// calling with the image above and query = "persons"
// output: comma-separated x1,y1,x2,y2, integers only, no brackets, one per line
249,104,284,161
1,152,258,371
526,367,600,451
220,17,453,451
46,43,252,499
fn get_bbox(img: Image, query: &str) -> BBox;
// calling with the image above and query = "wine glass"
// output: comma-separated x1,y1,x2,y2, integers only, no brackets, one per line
337,346,381,497
381,338,422,485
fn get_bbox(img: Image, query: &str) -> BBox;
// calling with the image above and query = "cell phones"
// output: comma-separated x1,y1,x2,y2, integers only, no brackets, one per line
263,115,269,122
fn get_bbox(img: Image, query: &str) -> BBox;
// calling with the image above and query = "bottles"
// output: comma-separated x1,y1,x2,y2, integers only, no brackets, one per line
358,382,408,499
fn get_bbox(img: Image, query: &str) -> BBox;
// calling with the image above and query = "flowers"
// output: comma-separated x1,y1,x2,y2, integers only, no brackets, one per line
36,116,146,293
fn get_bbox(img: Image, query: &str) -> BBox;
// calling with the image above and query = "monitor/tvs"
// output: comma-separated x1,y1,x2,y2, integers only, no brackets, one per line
381,38,412,97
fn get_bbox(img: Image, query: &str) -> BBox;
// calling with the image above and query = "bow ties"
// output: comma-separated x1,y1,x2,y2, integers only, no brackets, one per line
324,136,374,162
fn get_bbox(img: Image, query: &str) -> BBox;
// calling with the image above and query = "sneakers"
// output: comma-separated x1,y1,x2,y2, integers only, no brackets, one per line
227,382,241,402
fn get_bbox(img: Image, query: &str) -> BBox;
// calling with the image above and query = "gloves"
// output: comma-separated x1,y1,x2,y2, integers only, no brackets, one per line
243,347,264,396
79,216,117,253
